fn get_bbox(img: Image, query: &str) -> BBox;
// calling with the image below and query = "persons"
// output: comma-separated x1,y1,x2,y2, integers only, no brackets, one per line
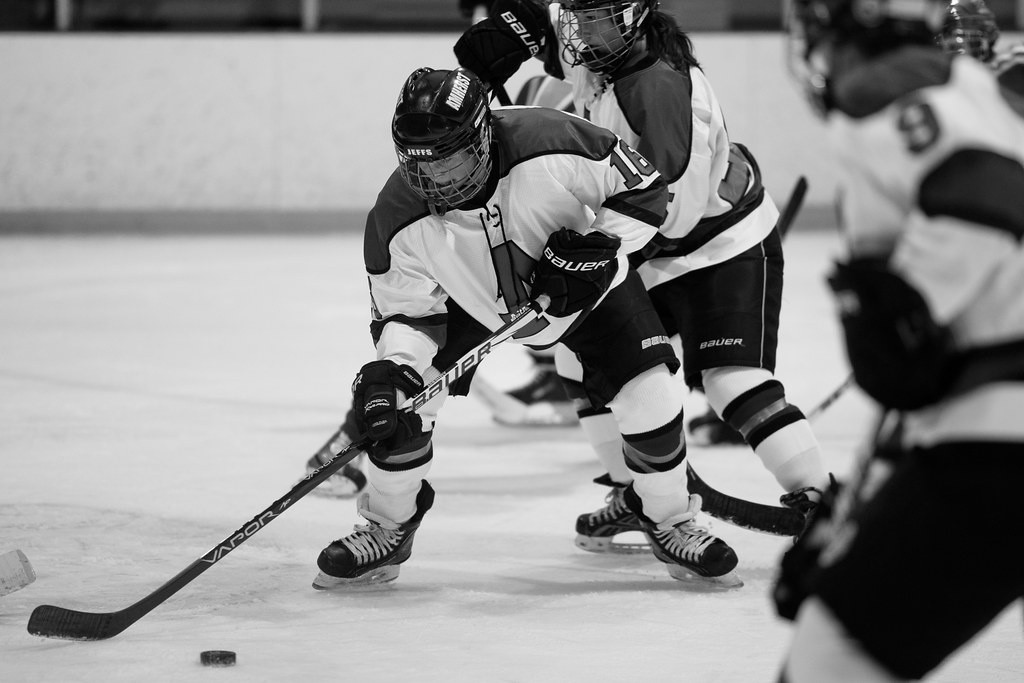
312,67,745,601
452,1,1023,682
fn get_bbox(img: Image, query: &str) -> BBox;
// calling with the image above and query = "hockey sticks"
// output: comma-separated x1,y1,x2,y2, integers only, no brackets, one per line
685,461,805,536
25,294,556,641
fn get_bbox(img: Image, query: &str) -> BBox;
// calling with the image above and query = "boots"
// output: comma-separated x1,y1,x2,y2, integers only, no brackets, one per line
497,352,581,426
624,481,745,590
573,472,656,561
312,480,437,589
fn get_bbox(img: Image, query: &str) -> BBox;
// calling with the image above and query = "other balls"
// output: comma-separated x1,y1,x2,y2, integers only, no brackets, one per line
202,650,234,666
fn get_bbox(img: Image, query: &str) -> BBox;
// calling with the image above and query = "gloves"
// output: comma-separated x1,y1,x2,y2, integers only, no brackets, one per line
352,360,427,461
531,229,622,317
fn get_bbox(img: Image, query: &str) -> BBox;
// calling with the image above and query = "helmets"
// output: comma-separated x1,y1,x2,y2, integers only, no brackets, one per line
391,66,490,162
793,0,946,107
936,1,1000,65
560,0,654,38
